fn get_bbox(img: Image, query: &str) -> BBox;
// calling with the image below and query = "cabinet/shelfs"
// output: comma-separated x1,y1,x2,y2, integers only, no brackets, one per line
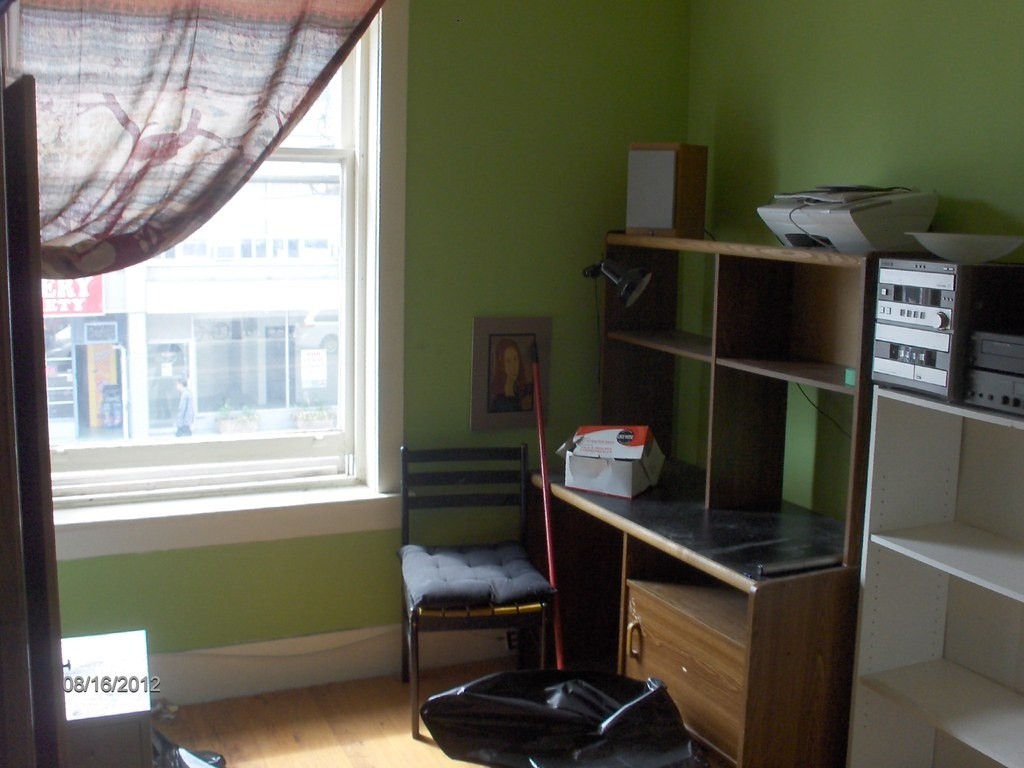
845,377,1024,768
525,229,877,767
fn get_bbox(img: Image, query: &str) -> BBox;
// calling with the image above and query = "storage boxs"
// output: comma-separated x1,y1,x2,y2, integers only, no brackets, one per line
558,423,665,497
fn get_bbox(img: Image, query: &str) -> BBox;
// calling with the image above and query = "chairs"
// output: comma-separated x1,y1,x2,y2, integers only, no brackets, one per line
395,445,558,739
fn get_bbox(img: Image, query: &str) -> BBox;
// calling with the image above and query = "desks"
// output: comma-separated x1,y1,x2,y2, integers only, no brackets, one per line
61,626,155,767
